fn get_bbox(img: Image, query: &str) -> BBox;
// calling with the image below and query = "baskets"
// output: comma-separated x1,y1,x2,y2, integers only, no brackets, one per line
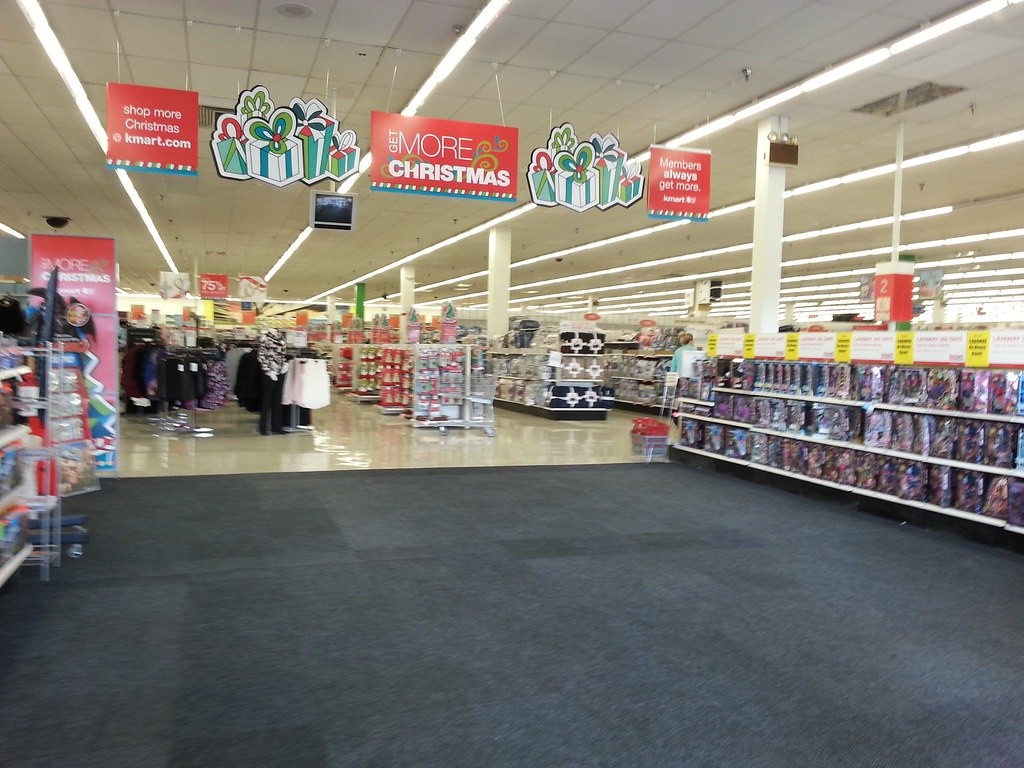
630,432,669,456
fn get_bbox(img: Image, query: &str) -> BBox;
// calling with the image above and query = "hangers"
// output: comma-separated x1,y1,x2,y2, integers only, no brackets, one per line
131,329,298,367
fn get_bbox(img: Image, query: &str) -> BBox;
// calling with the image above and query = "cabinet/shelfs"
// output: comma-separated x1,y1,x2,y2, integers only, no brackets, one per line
303,317,1024,539
0,342,63,591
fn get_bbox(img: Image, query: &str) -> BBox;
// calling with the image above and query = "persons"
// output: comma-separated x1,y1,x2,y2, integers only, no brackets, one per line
670,333,699,387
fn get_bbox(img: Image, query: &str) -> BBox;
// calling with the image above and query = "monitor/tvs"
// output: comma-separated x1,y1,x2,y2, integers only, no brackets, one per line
310,190,357,231
